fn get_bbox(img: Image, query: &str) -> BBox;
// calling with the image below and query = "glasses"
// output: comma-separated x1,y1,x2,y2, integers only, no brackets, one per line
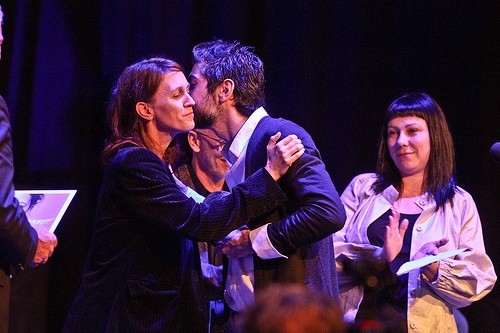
195,131,226,149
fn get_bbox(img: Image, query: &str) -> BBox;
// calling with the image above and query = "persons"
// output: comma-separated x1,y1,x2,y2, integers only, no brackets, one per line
62,58,305,333
0,6,58,333
332,93,498,333
13,194,45,213
173,129,232,333
188,40,346,333
